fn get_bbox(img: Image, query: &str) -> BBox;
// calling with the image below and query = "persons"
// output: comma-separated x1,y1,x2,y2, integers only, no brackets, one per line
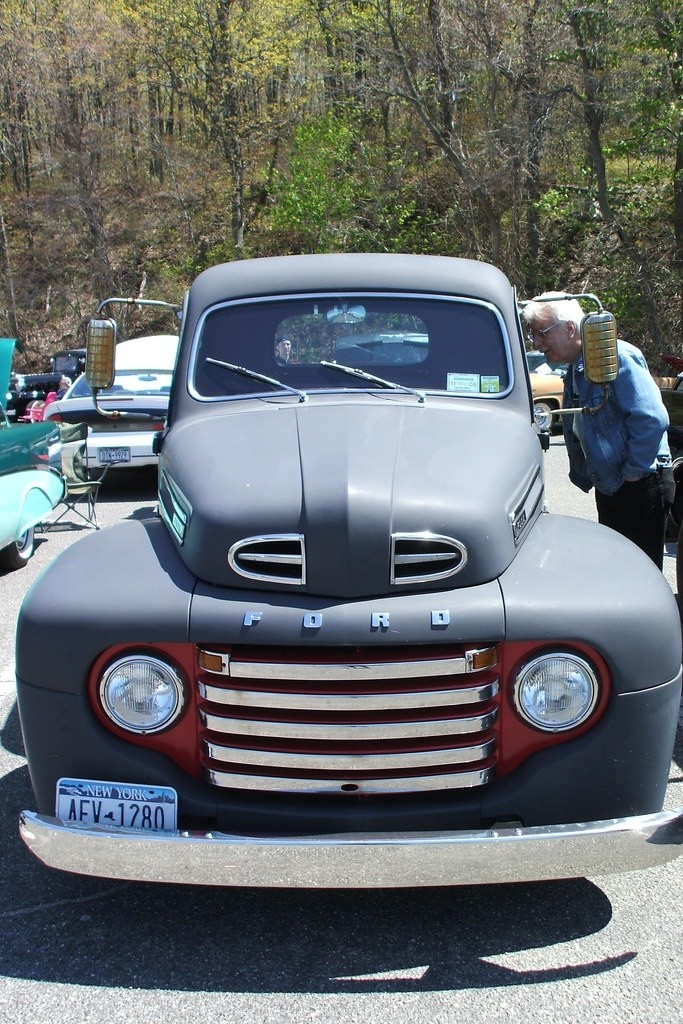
275,336,298,367
53,377,72,401
522,290,676,573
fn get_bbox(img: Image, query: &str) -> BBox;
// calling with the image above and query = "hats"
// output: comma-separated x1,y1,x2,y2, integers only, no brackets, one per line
275,334,290,346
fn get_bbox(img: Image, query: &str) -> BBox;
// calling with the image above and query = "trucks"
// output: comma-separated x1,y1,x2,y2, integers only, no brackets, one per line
525,351,677,433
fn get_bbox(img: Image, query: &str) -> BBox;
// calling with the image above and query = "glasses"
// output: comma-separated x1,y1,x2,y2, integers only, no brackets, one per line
528,323,560,343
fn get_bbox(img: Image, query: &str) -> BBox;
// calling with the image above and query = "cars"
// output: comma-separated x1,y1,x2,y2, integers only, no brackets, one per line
41,335,179,491
657,372,683,543
332,330,428,366
7,348,87,424
14,253,683,888
0,402,69,569
